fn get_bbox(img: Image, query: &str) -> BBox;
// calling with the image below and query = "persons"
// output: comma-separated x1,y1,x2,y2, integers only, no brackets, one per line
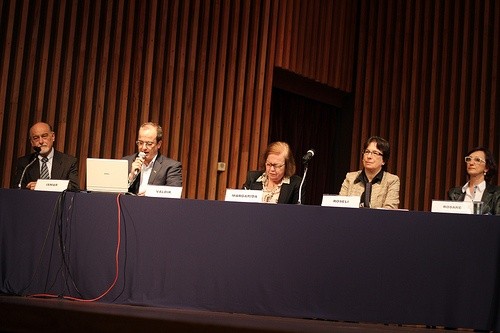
13,122,80,191
122,122,182,195
339,136,400,208
449,149,500,216
243,142,306,204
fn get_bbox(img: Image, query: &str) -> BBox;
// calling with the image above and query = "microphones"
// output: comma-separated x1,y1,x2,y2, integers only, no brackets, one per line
303,149,316,160
135,152,146,176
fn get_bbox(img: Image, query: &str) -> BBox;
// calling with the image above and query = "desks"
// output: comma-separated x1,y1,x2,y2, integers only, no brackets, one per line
0,187,500,331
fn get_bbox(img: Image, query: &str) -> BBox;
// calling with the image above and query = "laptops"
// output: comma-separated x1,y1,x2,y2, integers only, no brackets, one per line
86,158,136,196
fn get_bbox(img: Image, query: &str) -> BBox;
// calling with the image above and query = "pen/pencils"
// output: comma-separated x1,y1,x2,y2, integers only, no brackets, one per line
244,187,247,190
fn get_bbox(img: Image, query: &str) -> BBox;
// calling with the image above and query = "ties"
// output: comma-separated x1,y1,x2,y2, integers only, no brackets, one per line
40,158,50,179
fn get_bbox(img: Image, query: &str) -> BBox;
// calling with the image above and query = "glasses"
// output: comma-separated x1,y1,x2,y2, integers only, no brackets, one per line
465,156,486,164
135,141,157,146
363,150,383,156
265,162,285,169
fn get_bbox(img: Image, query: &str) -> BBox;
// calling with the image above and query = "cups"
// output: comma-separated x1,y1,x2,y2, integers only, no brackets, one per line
473,201,484,215
